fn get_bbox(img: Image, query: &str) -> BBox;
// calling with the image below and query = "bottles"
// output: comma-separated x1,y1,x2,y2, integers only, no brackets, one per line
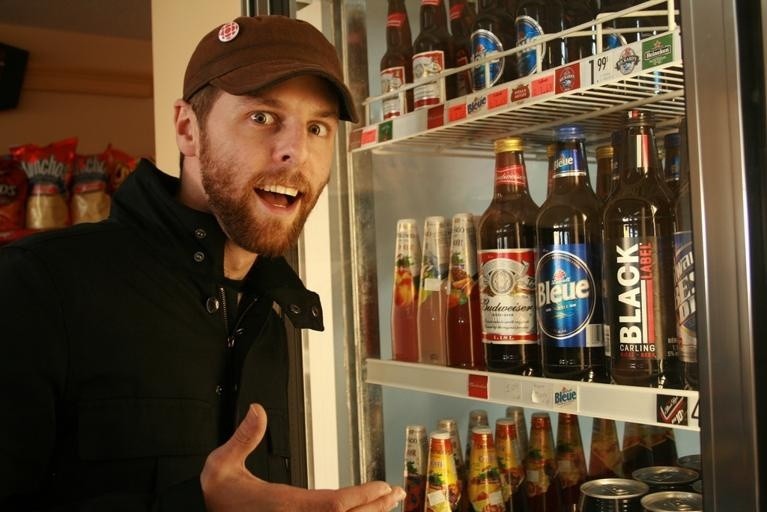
476,138,540,378
390,402,677,511
446,213,486,372
375,0,673,117
532,109,698,391
418,214,452,368
392,217,419,363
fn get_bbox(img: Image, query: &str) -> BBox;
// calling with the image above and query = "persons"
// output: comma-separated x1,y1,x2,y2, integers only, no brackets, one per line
1,12,408,512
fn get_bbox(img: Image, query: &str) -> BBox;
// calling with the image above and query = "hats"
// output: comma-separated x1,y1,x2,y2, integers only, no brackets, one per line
183,16,360,124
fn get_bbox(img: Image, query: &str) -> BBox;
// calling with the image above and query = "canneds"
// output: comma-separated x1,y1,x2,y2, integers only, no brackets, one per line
580,455,703,512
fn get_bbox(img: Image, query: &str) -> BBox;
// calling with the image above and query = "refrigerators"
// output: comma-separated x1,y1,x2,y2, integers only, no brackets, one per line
245,0,758,512
